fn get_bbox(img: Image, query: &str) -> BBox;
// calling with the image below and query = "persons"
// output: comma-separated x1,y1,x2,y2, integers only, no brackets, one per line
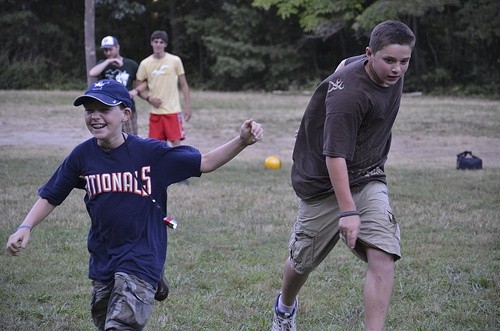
272,20,415,331
6,79,264,331
89,35,147,135
135,31,191,184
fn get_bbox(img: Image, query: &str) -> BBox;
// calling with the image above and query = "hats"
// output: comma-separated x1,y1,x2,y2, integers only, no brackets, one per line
73,79,132,108
101,36,119,49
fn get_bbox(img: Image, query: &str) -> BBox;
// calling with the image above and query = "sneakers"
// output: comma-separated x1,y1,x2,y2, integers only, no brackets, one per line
271,291,298,331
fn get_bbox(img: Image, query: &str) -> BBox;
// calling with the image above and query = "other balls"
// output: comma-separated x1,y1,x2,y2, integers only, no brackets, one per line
264,156,280,169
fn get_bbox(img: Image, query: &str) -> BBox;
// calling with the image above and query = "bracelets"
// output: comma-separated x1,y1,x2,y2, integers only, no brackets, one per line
146,94,151,102
338,210,361,218
135,87,140,95
17,225,32,232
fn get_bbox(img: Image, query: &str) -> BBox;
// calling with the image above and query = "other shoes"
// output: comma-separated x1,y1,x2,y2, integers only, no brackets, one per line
154,265,168,301
